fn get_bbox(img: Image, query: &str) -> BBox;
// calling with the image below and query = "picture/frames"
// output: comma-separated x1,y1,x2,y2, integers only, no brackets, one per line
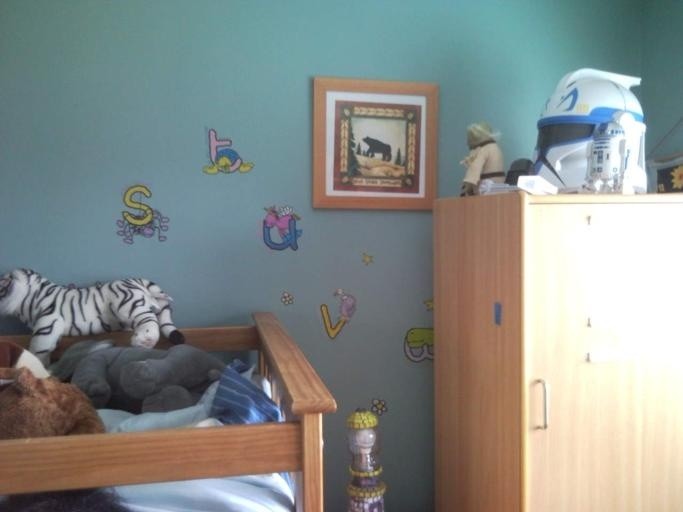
312,76,438,211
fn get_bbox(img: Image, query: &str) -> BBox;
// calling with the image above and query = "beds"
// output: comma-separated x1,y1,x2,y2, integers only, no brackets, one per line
0,310,341,512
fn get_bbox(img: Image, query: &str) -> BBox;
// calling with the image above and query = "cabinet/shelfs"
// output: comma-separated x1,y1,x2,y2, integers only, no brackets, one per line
432,189,682,510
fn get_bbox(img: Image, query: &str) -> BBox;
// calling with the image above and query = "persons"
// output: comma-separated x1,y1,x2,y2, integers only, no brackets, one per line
0,480,138,512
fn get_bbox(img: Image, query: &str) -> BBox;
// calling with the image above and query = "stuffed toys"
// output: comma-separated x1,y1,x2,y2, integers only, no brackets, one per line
1,368,109,440
1,268,185,368
49,339,231,414
